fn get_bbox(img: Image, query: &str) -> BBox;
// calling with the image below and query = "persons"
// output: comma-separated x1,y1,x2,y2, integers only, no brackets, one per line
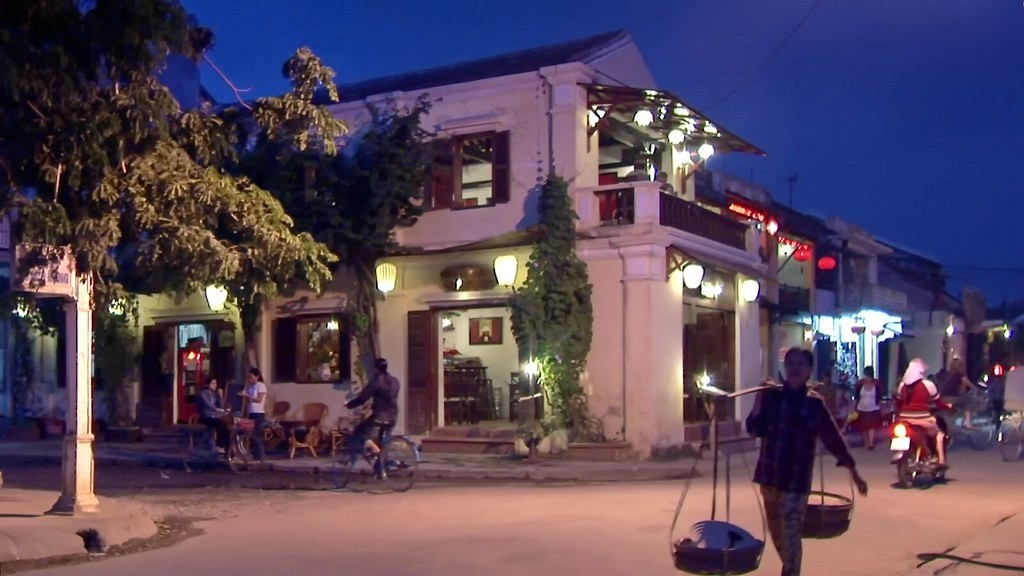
237,368,267,461
197,375,232,452
656,171,675,195
343,357,400,481
745,346,868,576
854,366,884,450
618,159,649,224
988,353,1024,445
895,357,953,469
927,358,980,430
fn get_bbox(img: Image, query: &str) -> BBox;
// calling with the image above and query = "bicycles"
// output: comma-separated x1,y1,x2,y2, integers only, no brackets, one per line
179,412,265,476
997,410,1024,462
934,389,994,451
331,404,420,492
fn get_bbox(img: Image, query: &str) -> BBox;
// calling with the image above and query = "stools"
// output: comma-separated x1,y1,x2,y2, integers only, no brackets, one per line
444,366,498,425
189,415,217,454
509,372,544,421
330,429,353,457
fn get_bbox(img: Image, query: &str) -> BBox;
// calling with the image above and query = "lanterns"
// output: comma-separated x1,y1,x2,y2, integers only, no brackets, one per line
792,249,812,262
818,256,836,269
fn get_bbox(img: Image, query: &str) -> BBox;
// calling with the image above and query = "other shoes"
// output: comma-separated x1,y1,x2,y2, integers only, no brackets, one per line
936,463,949,468
365,451,379,457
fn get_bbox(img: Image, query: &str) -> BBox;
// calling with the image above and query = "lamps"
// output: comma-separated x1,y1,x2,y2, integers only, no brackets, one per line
441,312,460,330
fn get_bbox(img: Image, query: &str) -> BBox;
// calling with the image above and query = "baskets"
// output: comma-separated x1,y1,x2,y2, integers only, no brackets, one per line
672,539,763,575
802,489,853,539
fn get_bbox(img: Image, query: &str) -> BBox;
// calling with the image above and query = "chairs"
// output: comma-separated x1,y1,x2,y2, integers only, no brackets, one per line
272,401,329,460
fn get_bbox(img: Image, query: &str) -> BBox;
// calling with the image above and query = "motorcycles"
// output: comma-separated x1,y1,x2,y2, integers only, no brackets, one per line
889,407,956,489
